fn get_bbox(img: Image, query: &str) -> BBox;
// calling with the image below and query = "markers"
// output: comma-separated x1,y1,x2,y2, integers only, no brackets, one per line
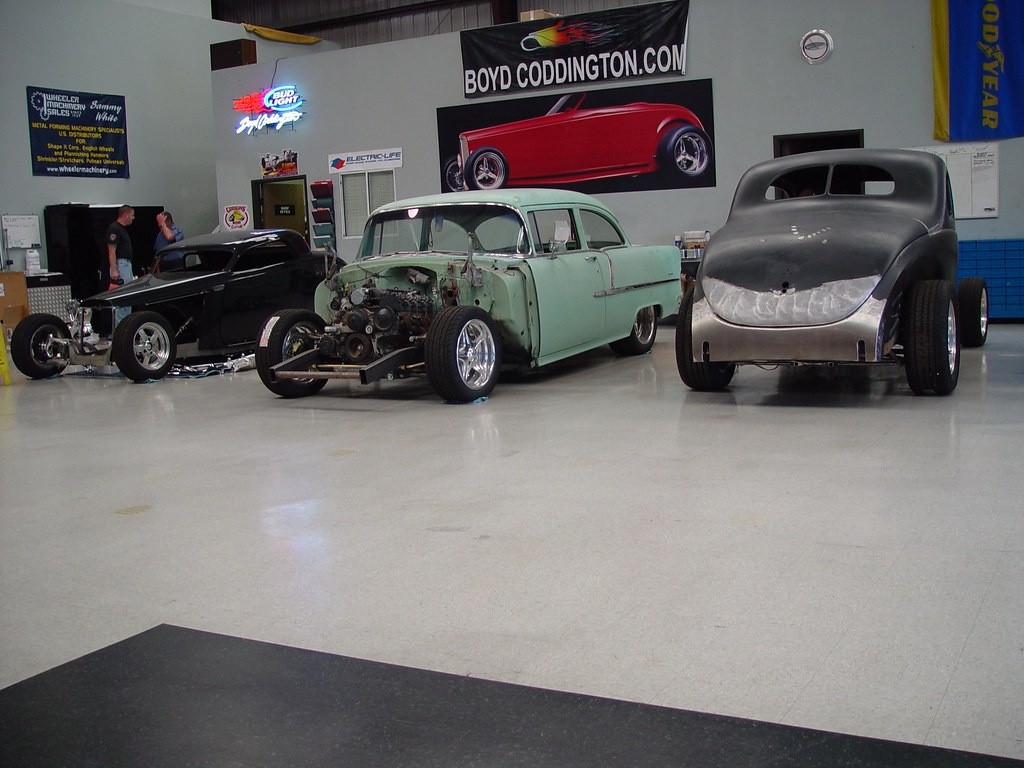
12,247,21,248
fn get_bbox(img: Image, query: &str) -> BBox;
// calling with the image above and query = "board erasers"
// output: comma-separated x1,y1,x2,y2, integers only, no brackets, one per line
31,243,40,247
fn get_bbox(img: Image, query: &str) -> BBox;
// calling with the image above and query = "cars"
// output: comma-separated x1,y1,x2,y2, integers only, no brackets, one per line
255,184,683,402
681,148,991,399
447,92,714,188
10,226,346,380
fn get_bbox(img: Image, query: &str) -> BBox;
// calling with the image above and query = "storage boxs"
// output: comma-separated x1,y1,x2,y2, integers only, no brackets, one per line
520,10,557,22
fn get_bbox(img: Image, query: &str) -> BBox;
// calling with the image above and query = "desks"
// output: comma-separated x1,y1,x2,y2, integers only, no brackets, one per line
26,274,73,324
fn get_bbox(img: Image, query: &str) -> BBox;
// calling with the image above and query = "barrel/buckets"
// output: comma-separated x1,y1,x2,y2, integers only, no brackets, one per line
26,249,40,270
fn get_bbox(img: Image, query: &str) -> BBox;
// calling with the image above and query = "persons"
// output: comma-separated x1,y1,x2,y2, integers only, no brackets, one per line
105,205,135,285
153,212,184,273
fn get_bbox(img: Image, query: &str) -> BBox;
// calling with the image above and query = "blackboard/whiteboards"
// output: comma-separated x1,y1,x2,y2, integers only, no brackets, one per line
1,214,42,250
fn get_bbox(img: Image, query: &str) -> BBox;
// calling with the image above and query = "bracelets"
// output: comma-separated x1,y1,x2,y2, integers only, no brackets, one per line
159,223,165,228
